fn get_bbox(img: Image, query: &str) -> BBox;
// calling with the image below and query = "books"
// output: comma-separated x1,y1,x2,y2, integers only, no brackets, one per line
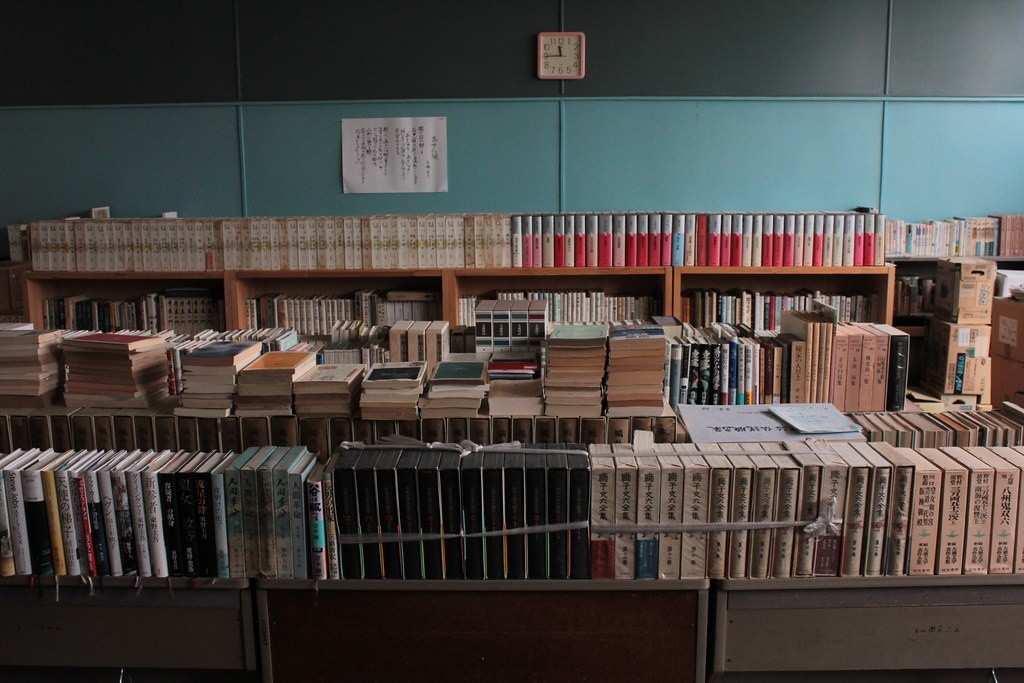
0,207,1023,580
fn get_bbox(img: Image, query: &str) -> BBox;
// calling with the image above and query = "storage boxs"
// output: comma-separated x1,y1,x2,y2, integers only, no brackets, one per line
921,317,991,395
990,353,1024,409
919,380,976,410
991,296,1024,363
907,384,944,412
935,258,997,325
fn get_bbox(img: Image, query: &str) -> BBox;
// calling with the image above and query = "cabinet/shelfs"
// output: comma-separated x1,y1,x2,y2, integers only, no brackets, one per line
0,257,1024,683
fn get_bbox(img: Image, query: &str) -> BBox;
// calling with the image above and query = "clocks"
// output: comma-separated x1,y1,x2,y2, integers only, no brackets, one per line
537,31,585,79
